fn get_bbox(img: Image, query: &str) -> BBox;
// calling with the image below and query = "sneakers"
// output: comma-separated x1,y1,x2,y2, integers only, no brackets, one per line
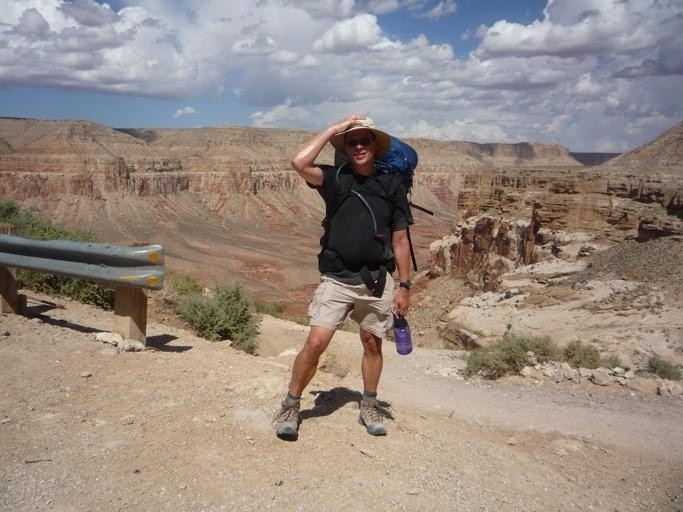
359,398,386,435
275,398,301,440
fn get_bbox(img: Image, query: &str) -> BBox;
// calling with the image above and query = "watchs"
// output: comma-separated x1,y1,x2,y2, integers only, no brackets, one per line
400,281,412,289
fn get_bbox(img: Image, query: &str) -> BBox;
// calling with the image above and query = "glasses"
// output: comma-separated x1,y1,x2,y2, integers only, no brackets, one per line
346,139,371,147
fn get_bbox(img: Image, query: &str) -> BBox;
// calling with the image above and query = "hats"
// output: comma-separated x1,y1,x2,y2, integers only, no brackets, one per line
330,117,391,156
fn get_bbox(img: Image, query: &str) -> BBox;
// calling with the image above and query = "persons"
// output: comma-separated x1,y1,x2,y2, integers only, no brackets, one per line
273,113,413,440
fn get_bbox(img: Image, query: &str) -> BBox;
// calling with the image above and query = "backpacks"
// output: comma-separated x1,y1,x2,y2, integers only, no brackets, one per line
319,136,417,230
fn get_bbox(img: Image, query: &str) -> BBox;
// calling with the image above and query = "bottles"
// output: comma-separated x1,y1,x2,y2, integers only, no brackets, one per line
392,311,413,356
